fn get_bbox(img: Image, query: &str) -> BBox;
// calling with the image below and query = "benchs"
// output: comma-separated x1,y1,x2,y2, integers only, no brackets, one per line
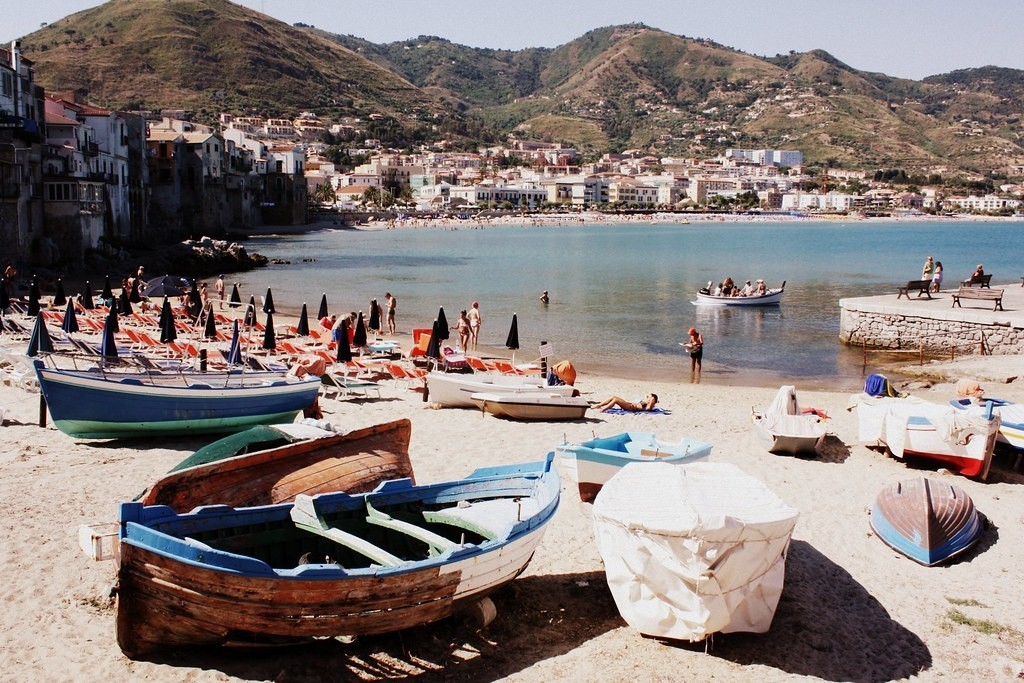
896,279,932,300
961,274,992,289
951,286,1004,312
296,522,406,568
366,515,458,552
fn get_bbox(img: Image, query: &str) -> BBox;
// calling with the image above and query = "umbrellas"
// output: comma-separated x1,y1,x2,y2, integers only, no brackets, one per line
0,271,141,368
141,275,188,297
159,283,328,364
505,312,520,366
337,300,380,365
426,305,449,359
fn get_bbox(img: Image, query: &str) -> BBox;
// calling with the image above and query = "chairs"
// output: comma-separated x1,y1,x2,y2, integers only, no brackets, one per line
0,298,547,406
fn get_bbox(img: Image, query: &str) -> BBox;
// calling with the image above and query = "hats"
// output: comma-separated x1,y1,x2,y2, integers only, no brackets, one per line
139,266,144,269
219,274,225,278
472,301,478,307
688,328,695,335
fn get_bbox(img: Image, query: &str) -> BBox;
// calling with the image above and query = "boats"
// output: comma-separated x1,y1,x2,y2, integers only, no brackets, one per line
750,387,828,453
425,372,576,406
31,359,323,439
846,390,1002,475
696,288,783,305
869,476,987,566
558,429,715,502
469,393,591,421
595,463,800,638
115,452,562,664
138,419,416,514
950,396,1024,451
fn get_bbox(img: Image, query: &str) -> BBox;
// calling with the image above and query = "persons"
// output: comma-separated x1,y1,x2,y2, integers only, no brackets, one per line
921,256,934,280
932,261,943,292
707,277,767,297
467,302,481,345
540,291,549,303
964,264,984,282
128,266,144,289
450,309,475,352
383,218,585,231
688,327,703,372
369,292,396,335
593,393,658,414
215,274,225,309
201,283,208,304
183,281,205,323
327,311,357,341
